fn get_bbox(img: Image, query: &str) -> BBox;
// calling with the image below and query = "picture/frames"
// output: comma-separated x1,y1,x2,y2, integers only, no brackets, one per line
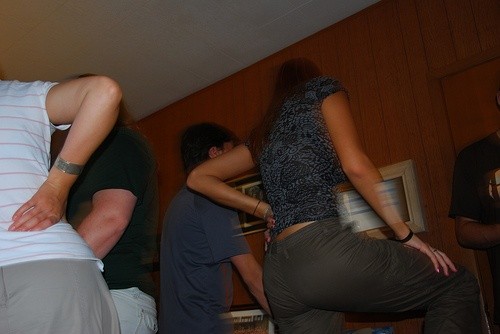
229,303,279,334
222,172,267,238
334,159,426,240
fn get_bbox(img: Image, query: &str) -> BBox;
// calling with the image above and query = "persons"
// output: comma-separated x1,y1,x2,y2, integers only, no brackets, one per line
450,86,500,334
1,72,158,334
159,121,271,334
187,57,480,334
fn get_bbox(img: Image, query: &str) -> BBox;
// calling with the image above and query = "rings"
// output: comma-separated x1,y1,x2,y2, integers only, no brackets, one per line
432,250,437,254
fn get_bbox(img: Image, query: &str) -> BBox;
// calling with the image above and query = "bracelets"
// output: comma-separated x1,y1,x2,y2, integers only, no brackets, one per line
252,199,275,221
54,155,84,175
393,228,413,242
